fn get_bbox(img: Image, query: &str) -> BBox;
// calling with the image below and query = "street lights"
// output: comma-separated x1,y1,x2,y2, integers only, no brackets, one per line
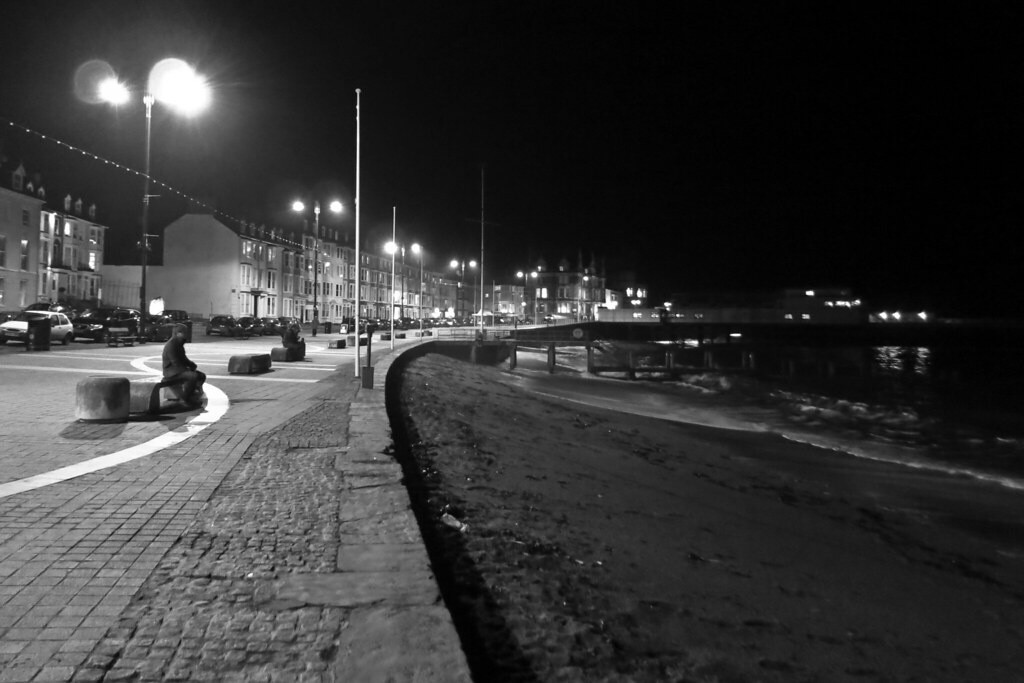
384,242,420,330
517,271,538,323
98,74,210,344
450,259,477,326
292,199,343,337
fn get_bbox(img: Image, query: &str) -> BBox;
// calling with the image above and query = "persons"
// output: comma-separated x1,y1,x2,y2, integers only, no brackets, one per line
161,323,207,397
311,316,320,337
280,320,306,361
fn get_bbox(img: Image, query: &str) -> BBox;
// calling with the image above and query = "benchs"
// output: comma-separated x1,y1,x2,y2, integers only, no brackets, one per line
270,347,297,361
396,333,406,338
330,338,346,350
228,352,272,375
382,334,392,340
129,377,183,415
360,338,367,345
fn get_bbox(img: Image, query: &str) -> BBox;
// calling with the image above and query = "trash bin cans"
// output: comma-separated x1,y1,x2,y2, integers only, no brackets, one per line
325,321,331,333
402,317,411,329
28,317,51,351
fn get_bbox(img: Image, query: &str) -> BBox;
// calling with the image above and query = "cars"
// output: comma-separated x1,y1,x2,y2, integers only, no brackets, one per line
0,303,193,347
359,312,554,329
206,315,302,340
